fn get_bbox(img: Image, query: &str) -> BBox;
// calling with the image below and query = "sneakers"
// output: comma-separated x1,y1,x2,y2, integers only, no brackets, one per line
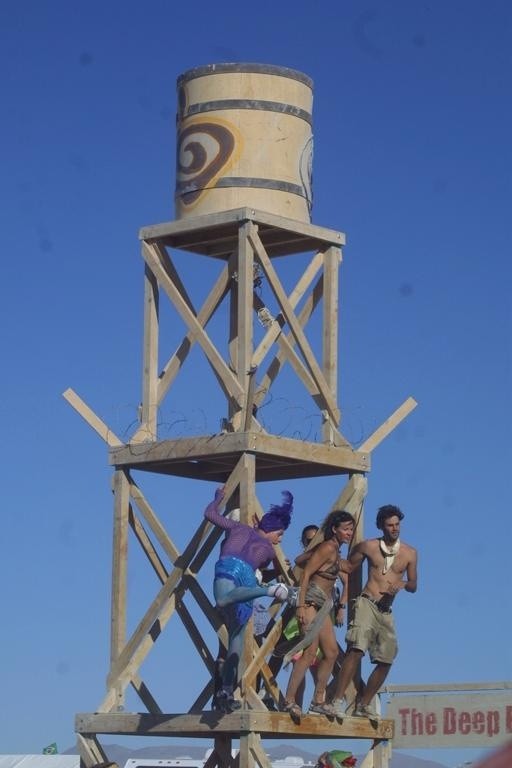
355,703,379,721
212,695,241,710
273,581,299,609
328,700,348,719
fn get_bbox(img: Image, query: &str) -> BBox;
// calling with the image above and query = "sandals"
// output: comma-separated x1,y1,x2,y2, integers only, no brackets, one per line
310,701,337,716
285,703,303,718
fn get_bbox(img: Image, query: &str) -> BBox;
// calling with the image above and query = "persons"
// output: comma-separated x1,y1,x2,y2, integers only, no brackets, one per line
205,485,418,721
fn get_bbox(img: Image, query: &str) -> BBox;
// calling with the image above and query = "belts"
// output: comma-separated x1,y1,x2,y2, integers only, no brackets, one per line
362,593,391,612
